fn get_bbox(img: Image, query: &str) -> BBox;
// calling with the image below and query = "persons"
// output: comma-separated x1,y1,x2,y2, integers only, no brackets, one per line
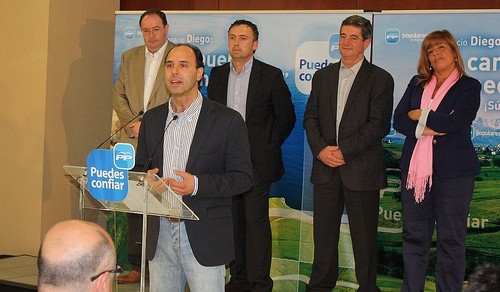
207,19,298,292
36,219,116,292
393,29,482,292
128,44,254,292
302,15,394,292
114,9,178,284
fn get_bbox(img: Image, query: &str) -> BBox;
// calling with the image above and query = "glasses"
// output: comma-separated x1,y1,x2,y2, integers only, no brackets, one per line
90,264,124,283
140,28,166,35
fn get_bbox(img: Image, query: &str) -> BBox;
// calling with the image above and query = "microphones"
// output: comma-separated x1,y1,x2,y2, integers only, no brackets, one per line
143,115,178,173
96,111,143,149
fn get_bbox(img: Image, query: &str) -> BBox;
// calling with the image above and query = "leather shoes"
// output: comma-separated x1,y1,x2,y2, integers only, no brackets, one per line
116,270,148,284
